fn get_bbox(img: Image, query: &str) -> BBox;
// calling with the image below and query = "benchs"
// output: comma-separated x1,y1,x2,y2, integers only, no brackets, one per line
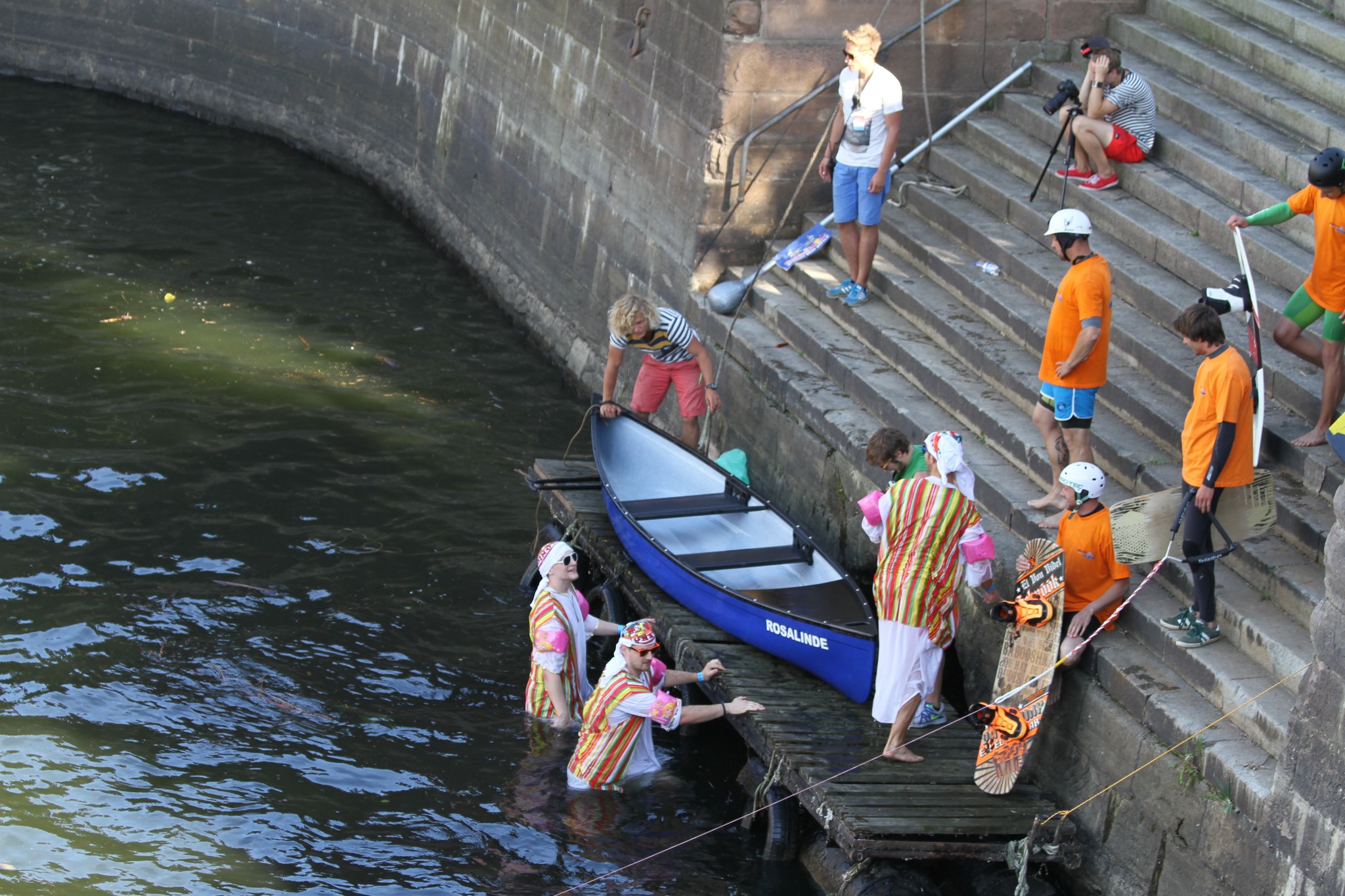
671,532,817,571
620,475,755,520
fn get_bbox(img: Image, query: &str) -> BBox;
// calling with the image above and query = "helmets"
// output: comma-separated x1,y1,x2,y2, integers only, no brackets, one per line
1044,209,1092,237
1309,148,1345,186
1058,461,1104,498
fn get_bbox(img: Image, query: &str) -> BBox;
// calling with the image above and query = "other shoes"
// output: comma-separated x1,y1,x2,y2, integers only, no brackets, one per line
1076,172,1120,191
1055,166,1094,182
1201,274,1252,317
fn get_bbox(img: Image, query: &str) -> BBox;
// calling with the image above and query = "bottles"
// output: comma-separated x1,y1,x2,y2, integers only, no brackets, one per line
974,261,1000,277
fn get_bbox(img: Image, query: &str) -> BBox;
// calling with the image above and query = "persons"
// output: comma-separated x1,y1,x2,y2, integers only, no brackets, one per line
524,541,645,729
1163,303,1255,650
1026,208,1112,514
1012,460,1133,706
1227,147,1345,446
1056,48,1155,190
568,619,769,794
863,428,948,728
598,294,721,452
862,426,1002,759
816,21,903,308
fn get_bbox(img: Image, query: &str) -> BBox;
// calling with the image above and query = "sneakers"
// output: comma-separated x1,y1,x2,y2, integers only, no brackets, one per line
825,278,854,298
968,701,1028,740
1175,619,1221,647
842,285,871,308
909,702,948,728
990,596,1054,629
1160,606,1201,630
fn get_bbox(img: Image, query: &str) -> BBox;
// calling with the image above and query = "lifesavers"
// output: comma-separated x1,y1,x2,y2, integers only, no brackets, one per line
665,679,710,737
520,529,580,587
583,586,628,652
742,785,796,862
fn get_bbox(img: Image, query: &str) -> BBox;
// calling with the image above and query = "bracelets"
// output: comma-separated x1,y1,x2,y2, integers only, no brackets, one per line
697,672,704,682
1091,80,1104,88
986,582,995,592
823,156,831,159
600,400,614,406
617,624,624,636
720,700,726,716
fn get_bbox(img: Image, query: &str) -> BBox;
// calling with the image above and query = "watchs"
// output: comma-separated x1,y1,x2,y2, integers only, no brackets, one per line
705,383,718,390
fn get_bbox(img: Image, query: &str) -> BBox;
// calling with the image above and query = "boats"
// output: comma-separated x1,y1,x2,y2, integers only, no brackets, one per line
588,390,880,702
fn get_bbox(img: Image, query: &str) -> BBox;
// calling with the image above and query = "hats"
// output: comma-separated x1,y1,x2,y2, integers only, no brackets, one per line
1080,37,1121,56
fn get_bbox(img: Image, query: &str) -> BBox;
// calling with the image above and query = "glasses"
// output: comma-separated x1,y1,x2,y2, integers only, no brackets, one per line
625,642,660,655
843,49,869,59
878,456,890,472
553,552,579,565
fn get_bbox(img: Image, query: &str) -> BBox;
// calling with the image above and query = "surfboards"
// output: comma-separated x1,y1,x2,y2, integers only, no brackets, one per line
974,538,1066,796
1109,470,1277,565
1233,224,1266,466
